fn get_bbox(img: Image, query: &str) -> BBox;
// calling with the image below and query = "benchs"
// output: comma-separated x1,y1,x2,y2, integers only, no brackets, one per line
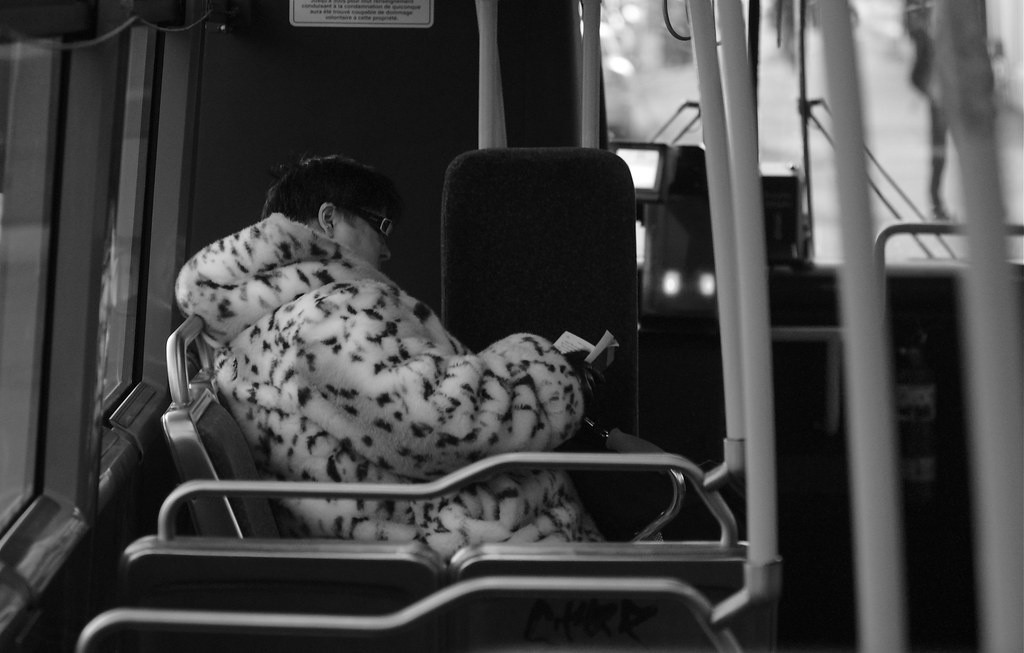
874,220,1023,390
75,317,750,652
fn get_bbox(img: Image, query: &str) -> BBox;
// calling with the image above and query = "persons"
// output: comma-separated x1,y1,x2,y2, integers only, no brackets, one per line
176,154,620,556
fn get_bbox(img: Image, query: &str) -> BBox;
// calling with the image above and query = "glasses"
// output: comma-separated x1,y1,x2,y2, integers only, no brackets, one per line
358,209,396,240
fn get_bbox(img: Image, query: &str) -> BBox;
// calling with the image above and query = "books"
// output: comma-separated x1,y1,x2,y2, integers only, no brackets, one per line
551,328,619,372
